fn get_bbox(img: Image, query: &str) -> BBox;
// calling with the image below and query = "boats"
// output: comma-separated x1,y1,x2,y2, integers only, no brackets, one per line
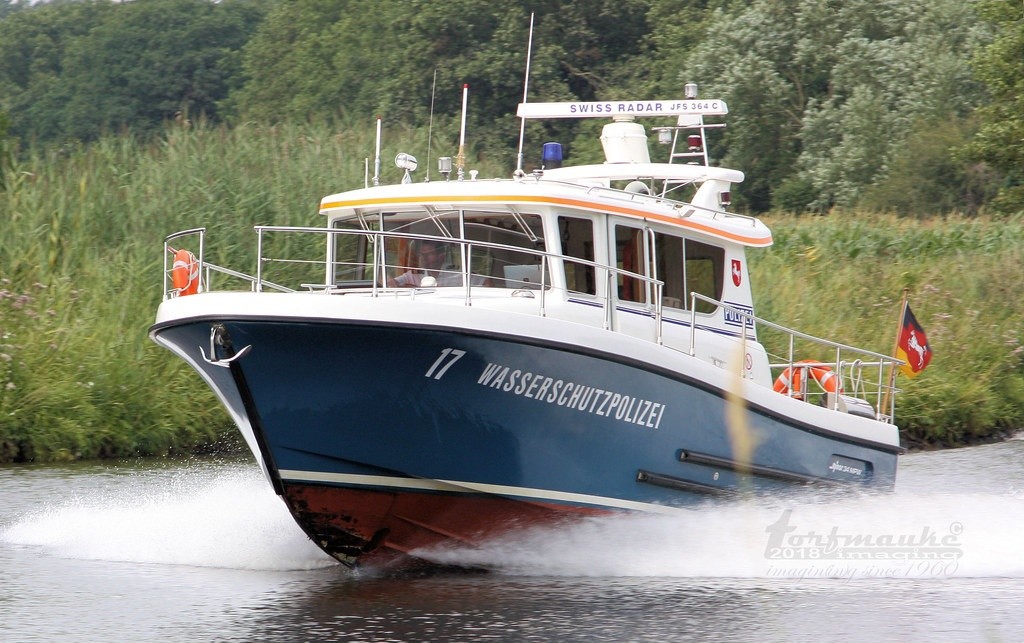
151,83,904,570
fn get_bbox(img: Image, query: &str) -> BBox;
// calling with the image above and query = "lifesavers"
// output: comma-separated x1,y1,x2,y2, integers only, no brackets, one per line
773,359,844,398
173,248,200,295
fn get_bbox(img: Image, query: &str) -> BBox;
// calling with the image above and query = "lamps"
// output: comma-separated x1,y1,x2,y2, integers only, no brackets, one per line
395,153,417,184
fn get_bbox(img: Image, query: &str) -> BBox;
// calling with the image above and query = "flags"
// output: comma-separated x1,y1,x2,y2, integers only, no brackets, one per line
893,302,934,380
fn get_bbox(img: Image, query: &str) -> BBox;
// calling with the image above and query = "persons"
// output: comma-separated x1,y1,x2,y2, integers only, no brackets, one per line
378,237,483,288
